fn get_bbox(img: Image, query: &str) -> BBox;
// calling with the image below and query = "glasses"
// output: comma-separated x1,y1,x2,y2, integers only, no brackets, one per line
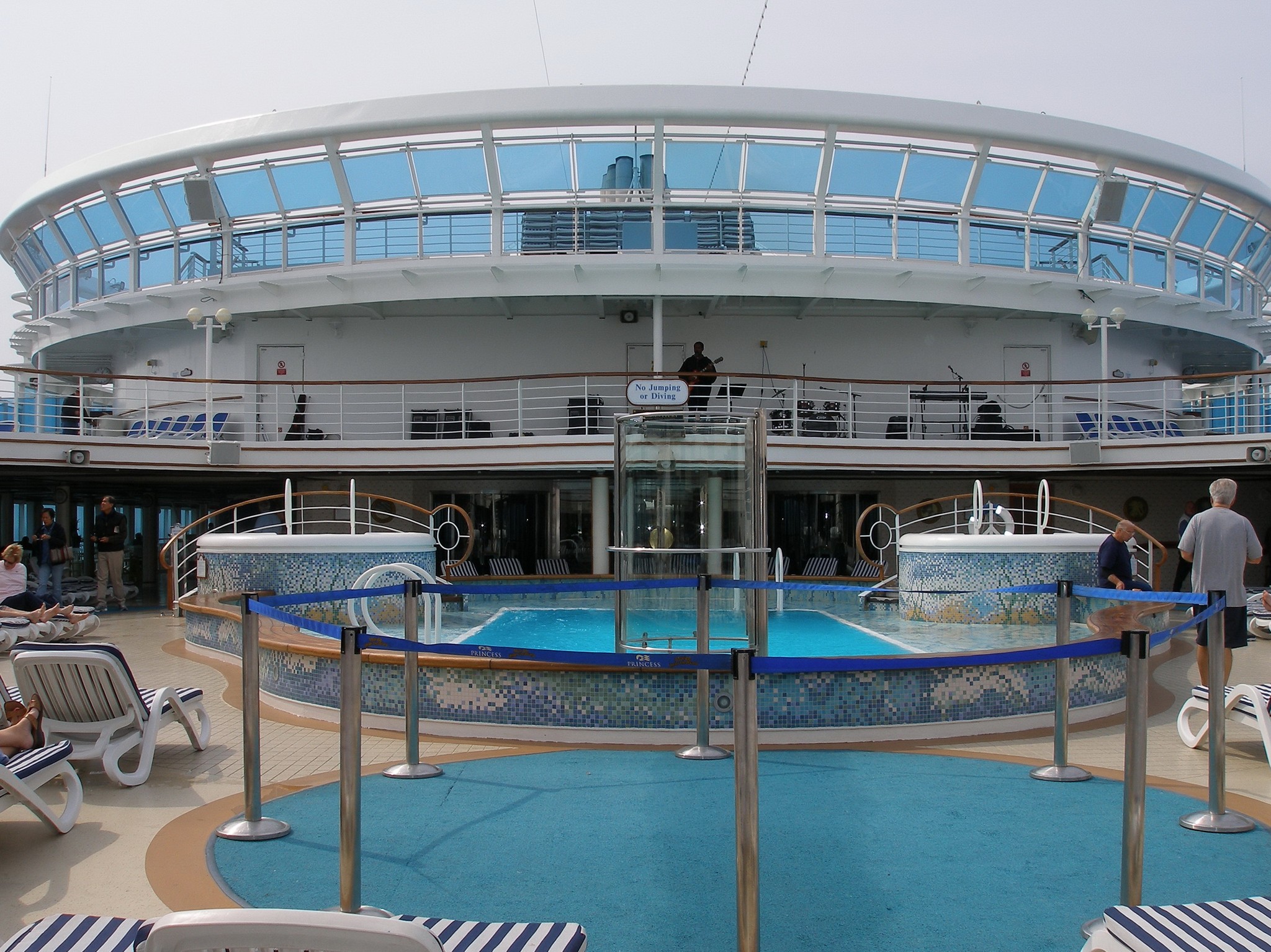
36,538,43,541
1123,530,1135,536
2,557,16,564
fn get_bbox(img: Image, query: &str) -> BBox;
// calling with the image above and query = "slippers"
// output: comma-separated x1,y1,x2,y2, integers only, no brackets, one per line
23,693,45,750
4,700,27,726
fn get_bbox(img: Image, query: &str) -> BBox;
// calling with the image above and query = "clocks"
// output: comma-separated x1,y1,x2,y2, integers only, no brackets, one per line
95,367,113,385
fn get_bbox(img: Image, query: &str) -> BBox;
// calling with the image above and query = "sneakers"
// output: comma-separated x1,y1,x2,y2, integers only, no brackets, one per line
94,604,108,612
119,604,128,611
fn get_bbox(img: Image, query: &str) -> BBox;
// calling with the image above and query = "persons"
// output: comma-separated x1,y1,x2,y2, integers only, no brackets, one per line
60,388,97,435
132,532,143,557
31,508,67,607
207,522,214,533
1261,590,1271,611
677,341,717,423
1098,519,1153,593
1177,477,1263,689
0,543,89,624
0,601,60,624
90,495,129,612
254,499,282,534
0,692,45,767
18,536,32,550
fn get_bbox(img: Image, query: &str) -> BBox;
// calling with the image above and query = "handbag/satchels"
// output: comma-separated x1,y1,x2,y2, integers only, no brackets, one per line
50,541,74,563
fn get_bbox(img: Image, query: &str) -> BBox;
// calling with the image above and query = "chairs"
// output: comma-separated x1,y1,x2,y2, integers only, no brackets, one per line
435,552,893,592
114,410,231,444
1175,583,1270,770
1073,409,1187,444
0,569,594,952
